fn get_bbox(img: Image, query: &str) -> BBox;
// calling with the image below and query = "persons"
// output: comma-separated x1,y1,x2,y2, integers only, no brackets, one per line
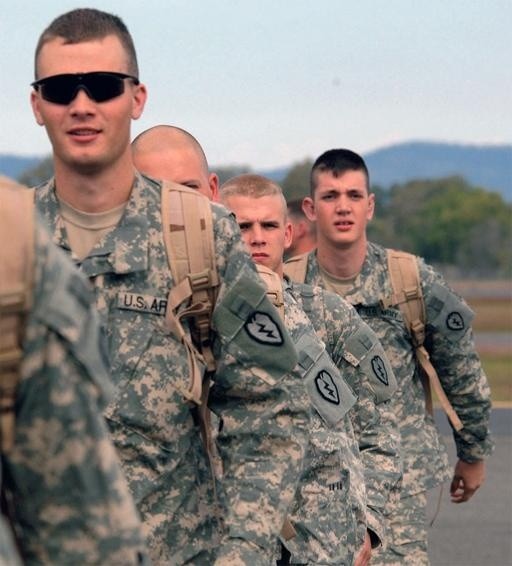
131,124,367,566
27,8,311,566
1,173,145,566
286,199,318,258
283,149,496,566
217,173,406,566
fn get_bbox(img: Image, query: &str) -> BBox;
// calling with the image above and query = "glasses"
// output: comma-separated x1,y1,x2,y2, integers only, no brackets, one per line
30,70,139,106
